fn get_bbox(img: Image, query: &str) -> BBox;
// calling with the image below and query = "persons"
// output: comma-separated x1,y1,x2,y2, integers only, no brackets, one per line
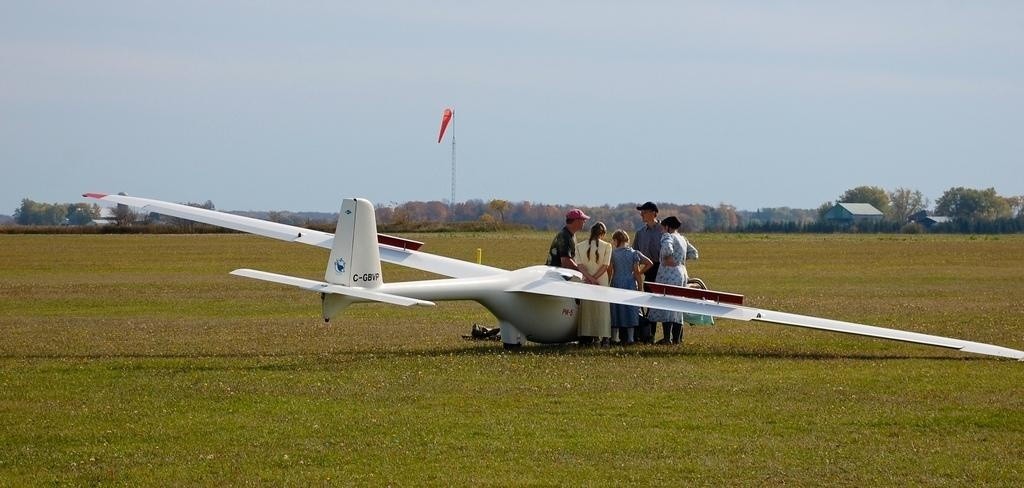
543,201,698,347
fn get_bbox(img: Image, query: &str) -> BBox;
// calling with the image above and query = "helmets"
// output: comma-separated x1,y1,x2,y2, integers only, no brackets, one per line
661,217,680,228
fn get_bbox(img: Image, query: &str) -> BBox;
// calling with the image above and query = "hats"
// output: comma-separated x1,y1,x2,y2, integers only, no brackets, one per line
567,209,590,220
637,202,658,211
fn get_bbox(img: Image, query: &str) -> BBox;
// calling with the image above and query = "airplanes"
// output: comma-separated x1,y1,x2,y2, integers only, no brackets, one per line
74,179,1024,368
